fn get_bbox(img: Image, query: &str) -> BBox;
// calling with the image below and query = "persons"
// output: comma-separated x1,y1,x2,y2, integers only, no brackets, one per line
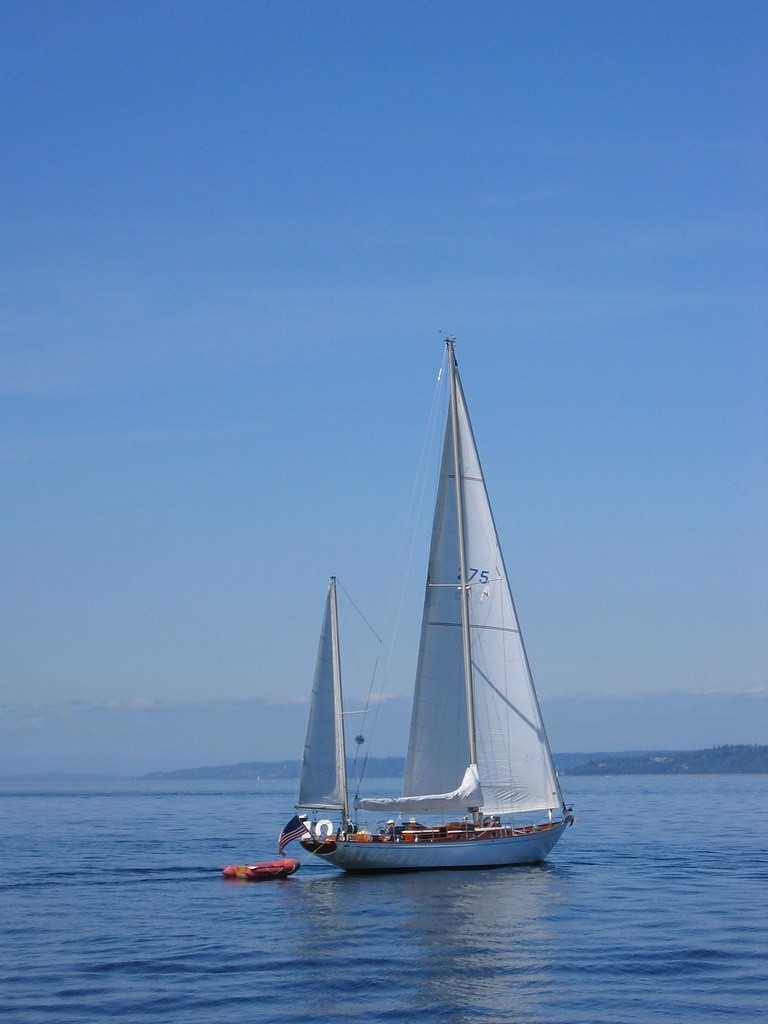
380,817,396,843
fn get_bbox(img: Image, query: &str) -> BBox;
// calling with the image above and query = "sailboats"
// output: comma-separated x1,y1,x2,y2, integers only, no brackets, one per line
296,334,576,872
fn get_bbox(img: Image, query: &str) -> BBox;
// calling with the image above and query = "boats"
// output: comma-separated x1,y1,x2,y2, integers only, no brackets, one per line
224,859,300,880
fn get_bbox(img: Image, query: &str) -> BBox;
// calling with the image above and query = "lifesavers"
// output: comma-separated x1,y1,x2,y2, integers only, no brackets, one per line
315,819,333,836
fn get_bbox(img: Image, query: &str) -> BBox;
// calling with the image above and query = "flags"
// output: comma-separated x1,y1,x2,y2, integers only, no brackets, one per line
279,816,309,856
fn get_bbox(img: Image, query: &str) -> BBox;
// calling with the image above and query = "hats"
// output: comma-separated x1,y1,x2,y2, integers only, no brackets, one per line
386,820,394,823
